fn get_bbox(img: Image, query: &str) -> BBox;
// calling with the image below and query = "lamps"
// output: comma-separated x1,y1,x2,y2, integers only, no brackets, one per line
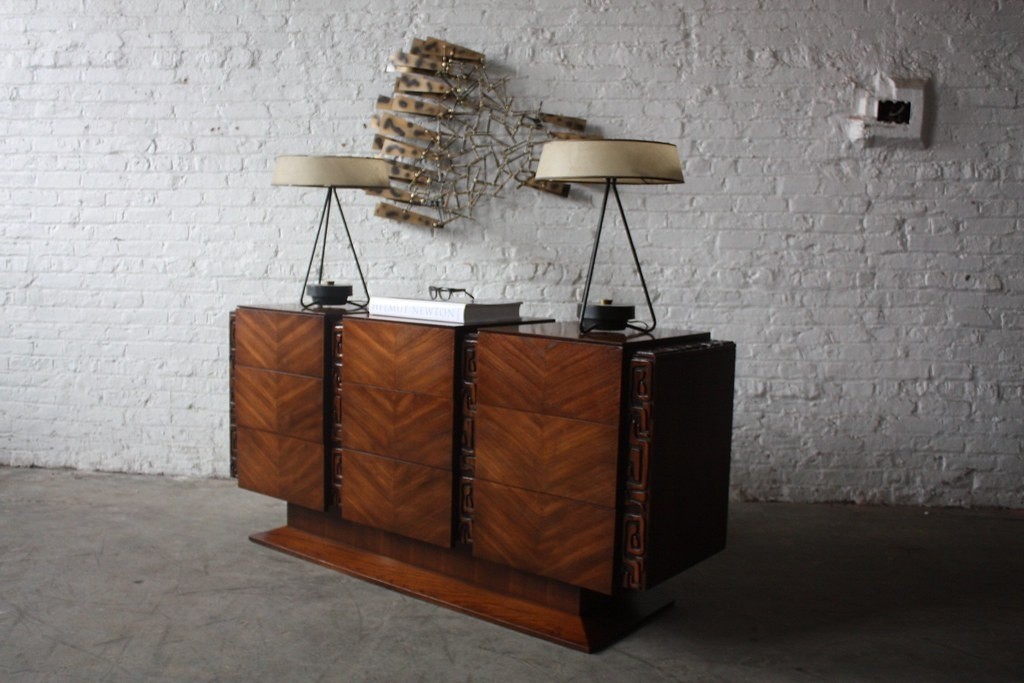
534,138,685,335
270,154,391,315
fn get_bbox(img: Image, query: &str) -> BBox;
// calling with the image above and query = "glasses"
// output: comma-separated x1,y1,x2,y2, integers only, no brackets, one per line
427,286,473,302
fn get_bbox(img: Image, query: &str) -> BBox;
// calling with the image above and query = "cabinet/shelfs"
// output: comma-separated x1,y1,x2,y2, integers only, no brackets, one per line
229,304,737,654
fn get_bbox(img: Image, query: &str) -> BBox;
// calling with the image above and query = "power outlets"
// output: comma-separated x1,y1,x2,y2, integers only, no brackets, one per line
851,75,929,147
